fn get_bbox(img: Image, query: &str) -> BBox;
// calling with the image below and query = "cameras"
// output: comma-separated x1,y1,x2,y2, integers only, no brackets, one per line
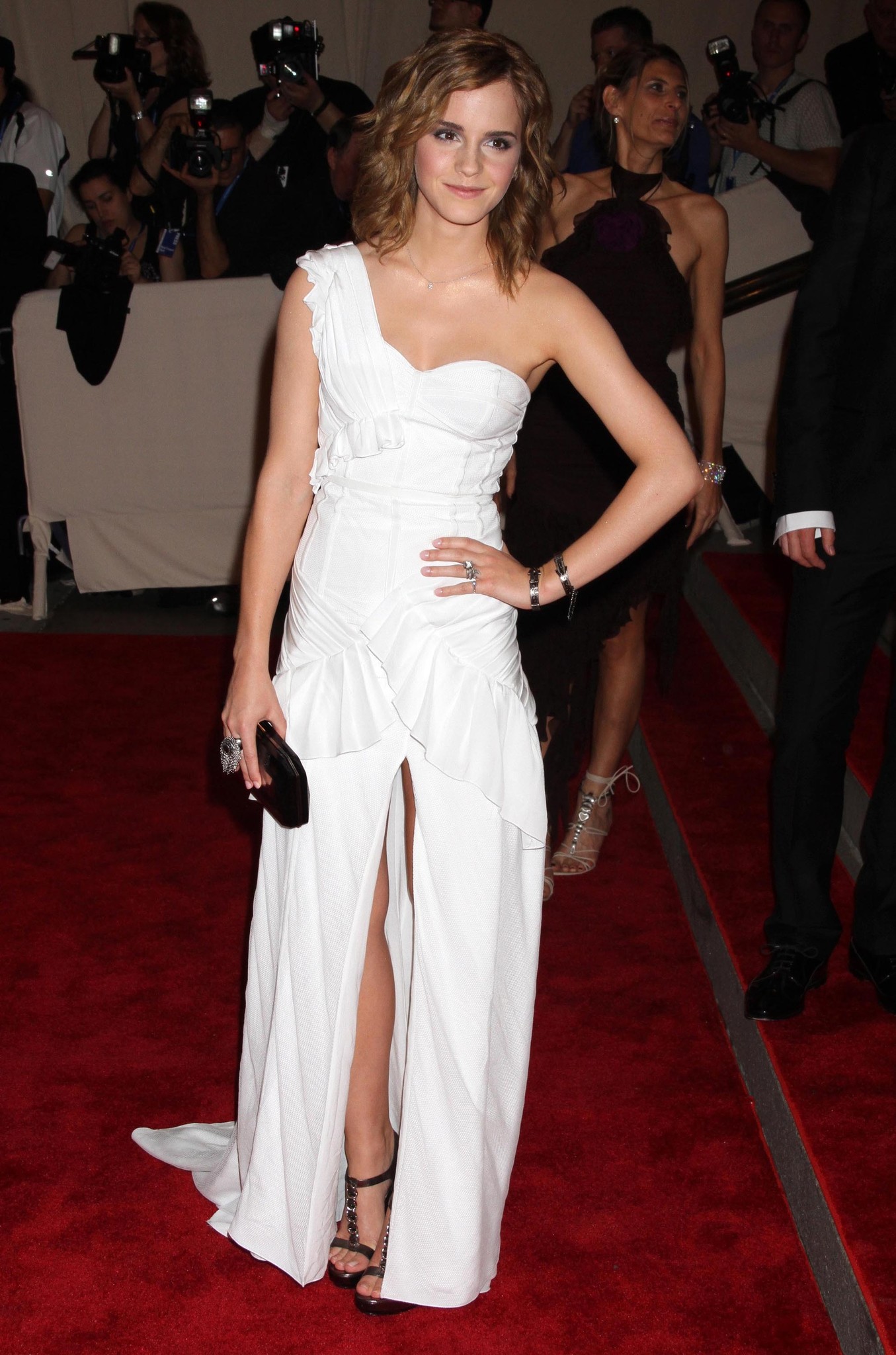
172,85,228,181
251,17,324,90
69,26,152,94
701,34,772,128
32,224,130,305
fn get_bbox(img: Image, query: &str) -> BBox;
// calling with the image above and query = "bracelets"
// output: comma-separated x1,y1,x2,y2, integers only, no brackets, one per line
527,567,542,611
554,553,579,620
698,460,727,485
311,94,330,121
129,108,146,123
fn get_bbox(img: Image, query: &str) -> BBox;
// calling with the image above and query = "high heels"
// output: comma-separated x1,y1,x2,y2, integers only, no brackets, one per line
355,1180,418,1316
329,1131,399,1288
551,764,640,875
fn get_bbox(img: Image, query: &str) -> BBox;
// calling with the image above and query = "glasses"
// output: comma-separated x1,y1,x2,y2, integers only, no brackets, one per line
135,36,161,46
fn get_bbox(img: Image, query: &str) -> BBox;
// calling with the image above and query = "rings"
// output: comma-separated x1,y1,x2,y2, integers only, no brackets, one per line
463,560,481,579
472,580,477,594
220,736,243,775
723,132,726,139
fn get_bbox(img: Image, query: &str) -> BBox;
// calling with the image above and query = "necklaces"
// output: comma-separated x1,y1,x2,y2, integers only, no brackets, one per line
610,173,664,204
403,239,500,289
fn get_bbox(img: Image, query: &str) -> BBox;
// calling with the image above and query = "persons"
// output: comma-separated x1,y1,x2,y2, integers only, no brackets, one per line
494,42,724,900
0,0,896,615
132,27,706,1317
743,121,896,1021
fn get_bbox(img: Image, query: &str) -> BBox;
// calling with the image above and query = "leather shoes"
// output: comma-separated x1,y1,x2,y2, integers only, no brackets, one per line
851,950,896,1014
744,947,828,1021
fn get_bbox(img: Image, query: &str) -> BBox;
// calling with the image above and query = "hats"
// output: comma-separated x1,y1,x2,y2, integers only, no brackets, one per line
251,18,324,64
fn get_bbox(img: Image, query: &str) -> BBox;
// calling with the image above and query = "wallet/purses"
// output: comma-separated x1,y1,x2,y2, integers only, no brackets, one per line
250,722,309,828
